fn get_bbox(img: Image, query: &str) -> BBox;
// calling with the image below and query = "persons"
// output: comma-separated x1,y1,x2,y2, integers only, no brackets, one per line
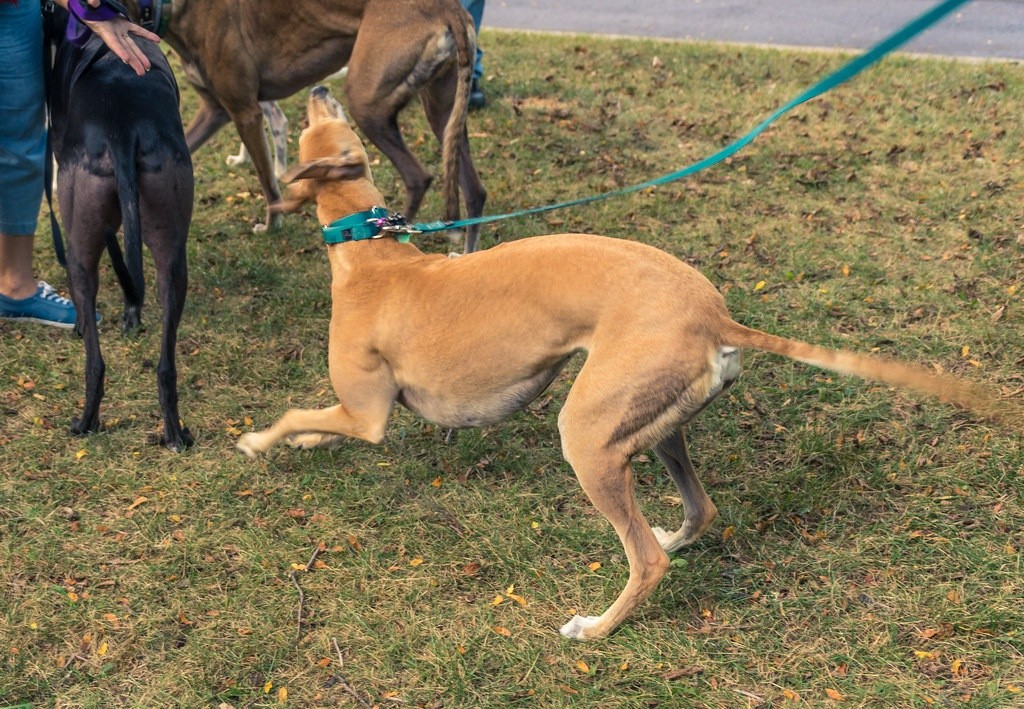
457,1,487,115
0,0,160,331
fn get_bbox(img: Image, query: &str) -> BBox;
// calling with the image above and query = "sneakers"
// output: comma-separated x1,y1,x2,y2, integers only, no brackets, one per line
0,281,102,328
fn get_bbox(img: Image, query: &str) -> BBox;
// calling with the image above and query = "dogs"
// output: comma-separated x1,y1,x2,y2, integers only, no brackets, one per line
135,0,489,257
41,0,194,450
235,85,1023,643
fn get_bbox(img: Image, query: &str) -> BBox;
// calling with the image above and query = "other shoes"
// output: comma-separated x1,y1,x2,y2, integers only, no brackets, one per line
466,76,487,113
